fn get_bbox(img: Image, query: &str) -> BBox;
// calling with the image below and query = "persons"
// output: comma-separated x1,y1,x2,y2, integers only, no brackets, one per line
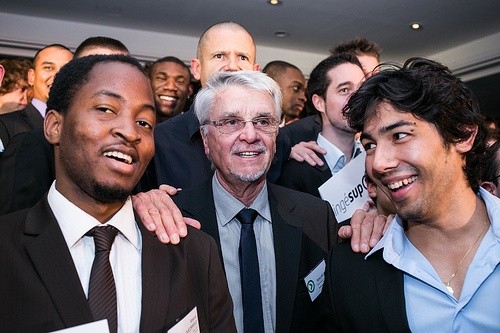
133,71,395,333
0,22,500,216
0,55,238,333
322,56,500,333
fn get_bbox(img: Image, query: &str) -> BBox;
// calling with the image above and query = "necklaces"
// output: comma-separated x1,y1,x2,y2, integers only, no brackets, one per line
403,224,485,297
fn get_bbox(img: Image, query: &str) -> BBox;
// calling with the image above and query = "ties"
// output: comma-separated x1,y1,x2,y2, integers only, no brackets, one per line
233,209,265,333
82,225,118,333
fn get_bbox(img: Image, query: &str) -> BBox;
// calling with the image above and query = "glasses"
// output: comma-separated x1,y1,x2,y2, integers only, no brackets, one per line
201,115,280,134
